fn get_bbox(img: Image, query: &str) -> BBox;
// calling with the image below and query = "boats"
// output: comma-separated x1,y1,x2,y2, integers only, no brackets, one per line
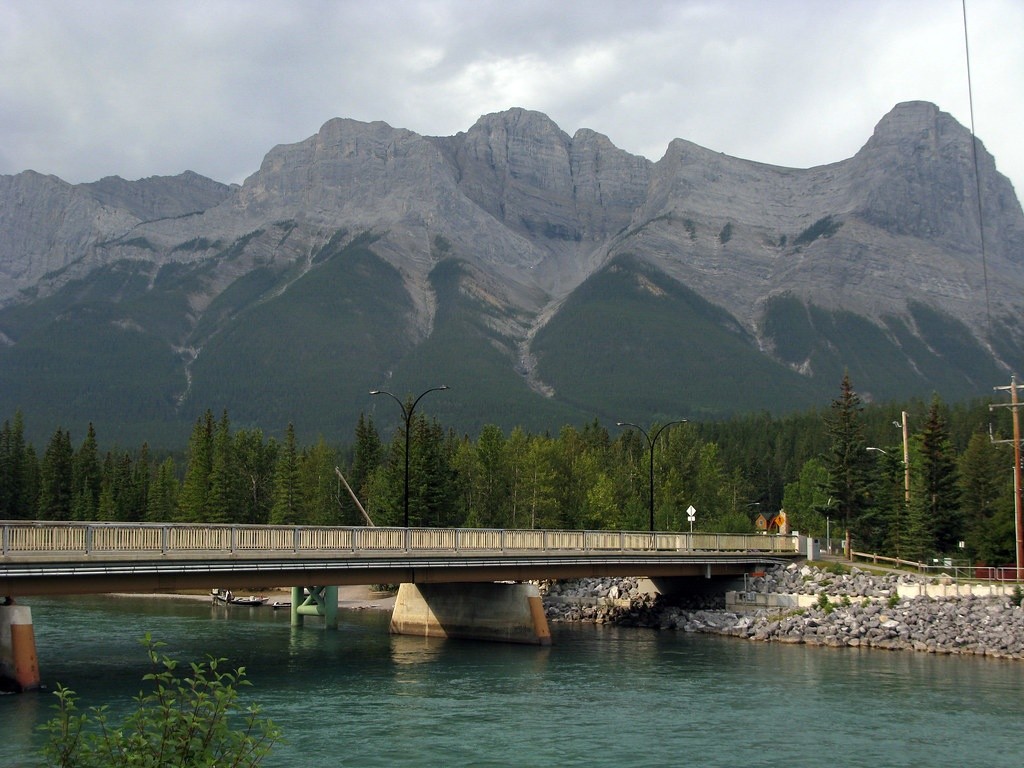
273,601,291,610
209,590,270,607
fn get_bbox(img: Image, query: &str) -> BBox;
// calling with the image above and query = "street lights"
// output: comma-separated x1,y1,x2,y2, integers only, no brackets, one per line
616,418,687,531
369,384,451,529
864,411,912,510
